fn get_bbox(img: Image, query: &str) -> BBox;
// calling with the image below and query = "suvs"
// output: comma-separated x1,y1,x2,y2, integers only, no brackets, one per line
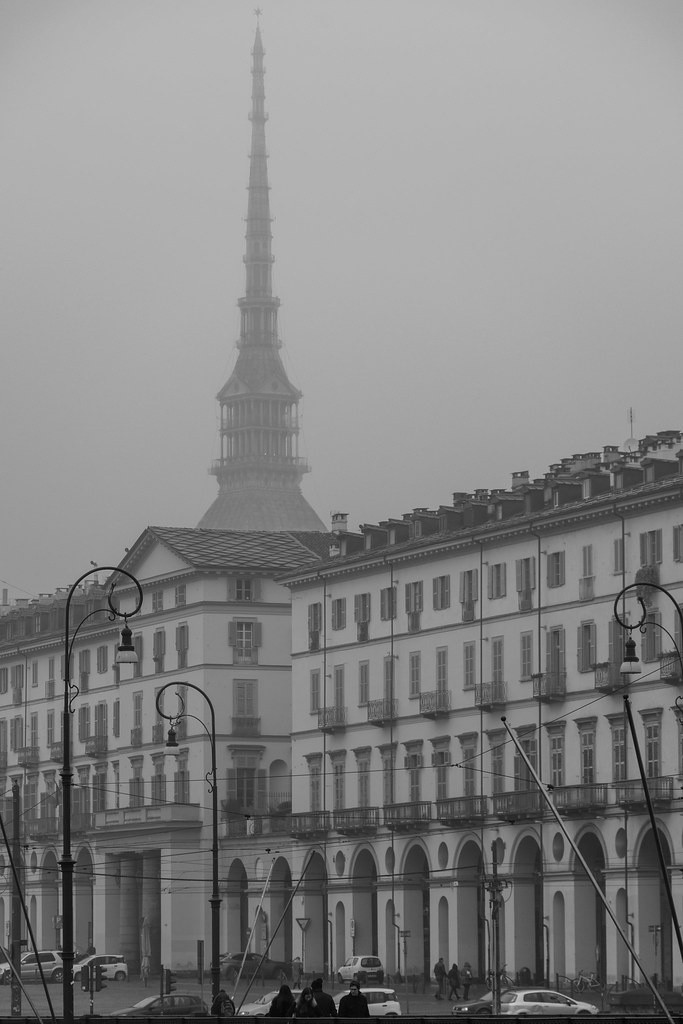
337,955,384,985
210,951,290,982
0,948,64,986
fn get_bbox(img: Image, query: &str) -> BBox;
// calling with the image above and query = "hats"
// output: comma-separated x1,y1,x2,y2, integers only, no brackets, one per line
311,978,323,989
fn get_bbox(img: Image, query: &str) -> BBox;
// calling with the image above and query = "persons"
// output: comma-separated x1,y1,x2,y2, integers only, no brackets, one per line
87,944,96,955
212,956,474,1018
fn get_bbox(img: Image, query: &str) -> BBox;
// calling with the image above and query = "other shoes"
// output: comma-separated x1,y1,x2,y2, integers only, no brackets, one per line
448,998,452,1001
456,997,461,1000
464,998,469,1001
439,997,444,1000
434,995,439,999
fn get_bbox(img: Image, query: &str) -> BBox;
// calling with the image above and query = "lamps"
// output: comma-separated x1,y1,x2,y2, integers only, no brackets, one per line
246,927,251,938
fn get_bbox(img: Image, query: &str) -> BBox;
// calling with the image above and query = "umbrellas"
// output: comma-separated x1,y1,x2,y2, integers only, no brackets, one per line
140,918,152,981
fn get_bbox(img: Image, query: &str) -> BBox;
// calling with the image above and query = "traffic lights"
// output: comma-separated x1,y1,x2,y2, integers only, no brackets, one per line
162,969,177,994
95,965,107,992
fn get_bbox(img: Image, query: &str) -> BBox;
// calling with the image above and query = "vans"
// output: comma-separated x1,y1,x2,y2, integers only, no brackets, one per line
332,988,402,1017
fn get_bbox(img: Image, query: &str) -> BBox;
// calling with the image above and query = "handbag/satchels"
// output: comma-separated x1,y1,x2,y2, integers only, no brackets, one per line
299,969,304,975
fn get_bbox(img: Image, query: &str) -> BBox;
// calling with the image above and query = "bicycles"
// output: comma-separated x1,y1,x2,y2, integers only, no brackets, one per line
570,969,606,993
485,964,515,991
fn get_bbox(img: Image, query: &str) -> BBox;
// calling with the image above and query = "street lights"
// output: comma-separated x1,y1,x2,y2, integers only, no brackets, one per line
57,566,143,1024
155,681,224,1003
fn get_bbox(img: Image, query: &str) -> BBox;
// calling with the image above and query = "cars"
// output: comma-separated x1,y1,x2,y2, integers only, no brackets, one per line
108,993,208,1017
451,987,518,1016
499,986,599,1016
73,954,129,982
234,989,303,1017
